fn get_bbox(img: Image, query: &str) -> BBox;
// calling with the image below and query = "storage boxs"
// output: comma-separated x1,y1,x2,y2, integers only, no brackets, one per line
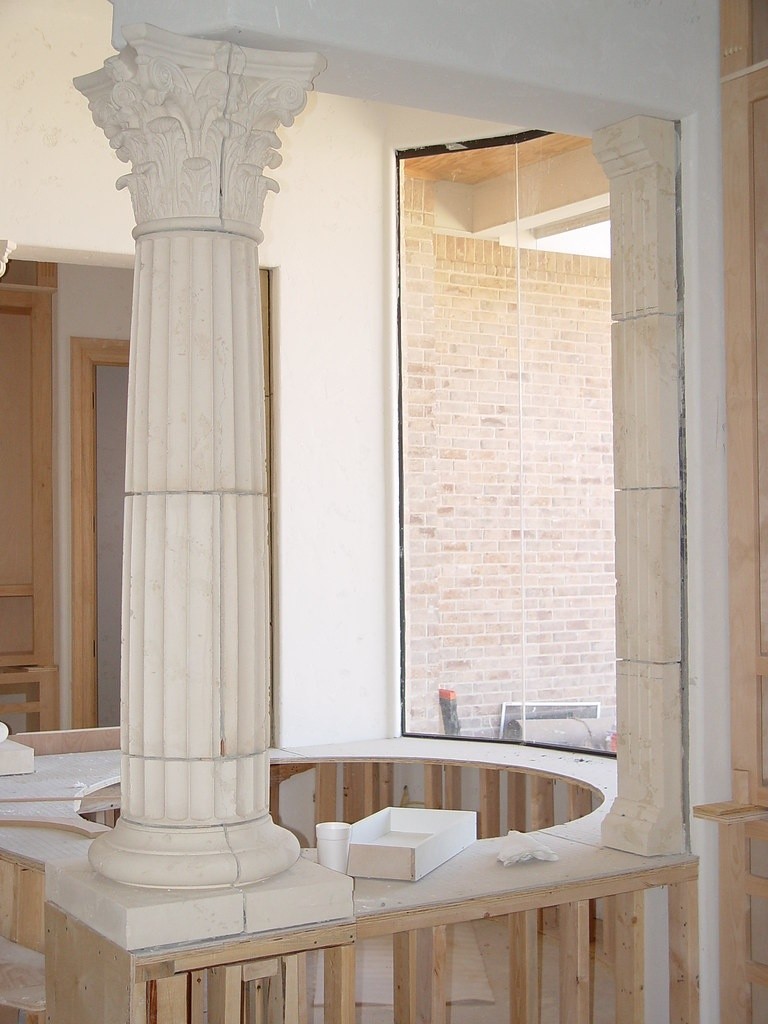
347,806,476,882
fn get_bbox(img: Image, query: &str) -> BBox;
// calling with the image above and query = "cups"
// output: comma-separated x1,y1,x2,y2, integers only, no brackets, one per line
315,822,352,875
